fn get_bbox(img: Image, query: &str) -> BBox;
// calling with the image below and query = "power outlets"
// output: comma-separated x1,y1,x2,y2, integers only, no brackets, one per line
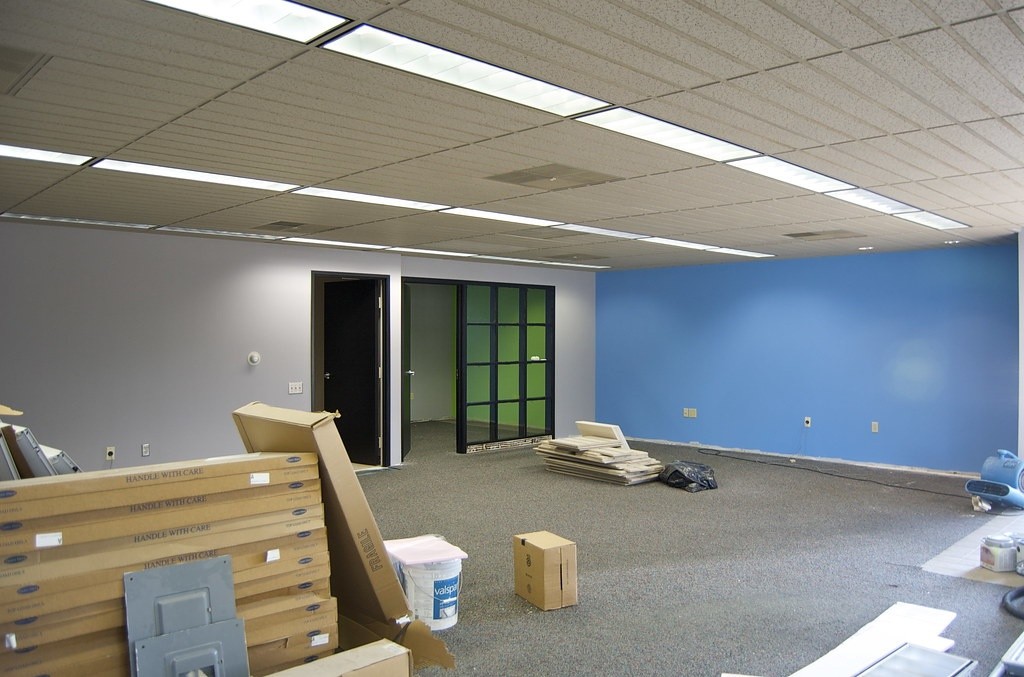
683,408,689,417
871,421,879,433
106,446,116,462
804,416,811,427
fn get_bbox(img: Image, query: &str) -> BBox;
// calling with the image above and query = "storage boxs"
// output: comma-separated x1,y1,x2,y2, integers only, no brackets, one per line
513,531,577,611
0,401,457,677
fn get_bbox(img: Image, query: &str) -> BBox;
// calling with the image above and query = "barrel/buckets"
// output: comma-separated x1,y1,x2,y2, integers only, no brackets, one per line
401,558,462,633
